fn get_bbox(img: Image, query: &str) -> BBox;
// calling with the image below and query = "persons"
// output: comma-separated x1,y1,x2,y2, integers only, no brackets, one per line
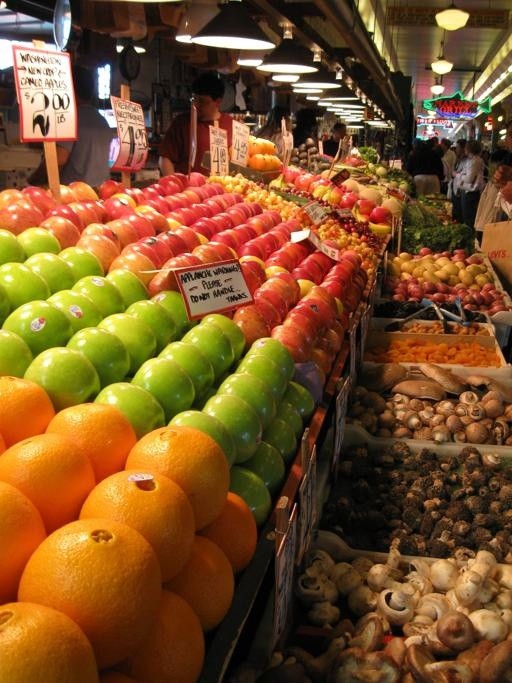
27,65,112,187
292,109,319,154
158,74,235,177
323,123,346,157
255,106,293,154
405,137,512,248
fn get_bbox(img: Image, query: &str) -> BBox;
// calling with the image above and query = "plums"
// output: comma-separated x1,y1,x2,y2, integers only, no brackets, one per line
387,278,508,316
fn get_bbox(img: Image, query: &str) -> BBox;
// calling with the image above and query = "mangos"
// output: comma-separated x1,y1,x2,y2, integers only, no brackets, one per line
388,252,490,291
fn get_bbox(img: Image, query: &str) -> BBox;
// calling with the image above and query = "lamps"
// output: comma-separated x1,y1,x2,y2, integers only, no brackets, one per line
436,0,471,30
157,0,360,101
431,40,454,75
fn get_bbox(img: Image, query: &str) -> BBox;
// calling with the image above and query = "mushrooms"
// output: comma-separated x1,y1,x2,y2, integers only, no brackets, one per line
258,362,512,683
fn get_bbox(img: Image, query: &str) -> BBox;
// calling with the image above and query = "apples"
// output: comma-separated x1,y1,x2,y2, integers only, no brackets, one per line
0,166,393,529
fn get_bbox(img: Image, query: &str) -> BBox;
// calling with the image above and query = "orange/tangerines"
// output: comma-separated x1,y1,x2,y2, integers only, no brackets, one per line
0,375,259,683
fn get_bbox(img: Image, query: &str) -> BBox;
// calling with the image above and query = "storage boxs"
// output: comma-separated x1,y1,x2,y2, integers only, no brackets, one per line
265,243,511,681
